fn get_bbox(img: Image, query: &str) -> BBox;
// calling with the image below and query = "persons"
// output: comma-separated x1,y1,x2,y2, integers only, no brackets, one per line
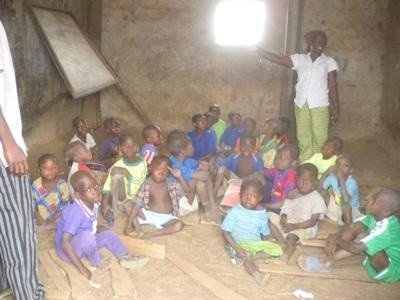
65,104,365,264
220,175,286,289
0,17,46,300
54,170,150,283
298,187,400,284
255,29,339,162
31,154,73,231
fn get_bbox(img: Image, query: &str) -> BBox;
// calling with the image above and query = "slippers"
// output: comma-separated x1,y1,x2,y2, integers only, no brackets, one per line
297,255,336,273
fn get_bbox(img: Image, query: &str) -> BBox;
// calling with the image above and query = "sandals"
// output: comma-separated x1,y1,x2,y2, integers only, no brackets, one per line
117,253,149,269
